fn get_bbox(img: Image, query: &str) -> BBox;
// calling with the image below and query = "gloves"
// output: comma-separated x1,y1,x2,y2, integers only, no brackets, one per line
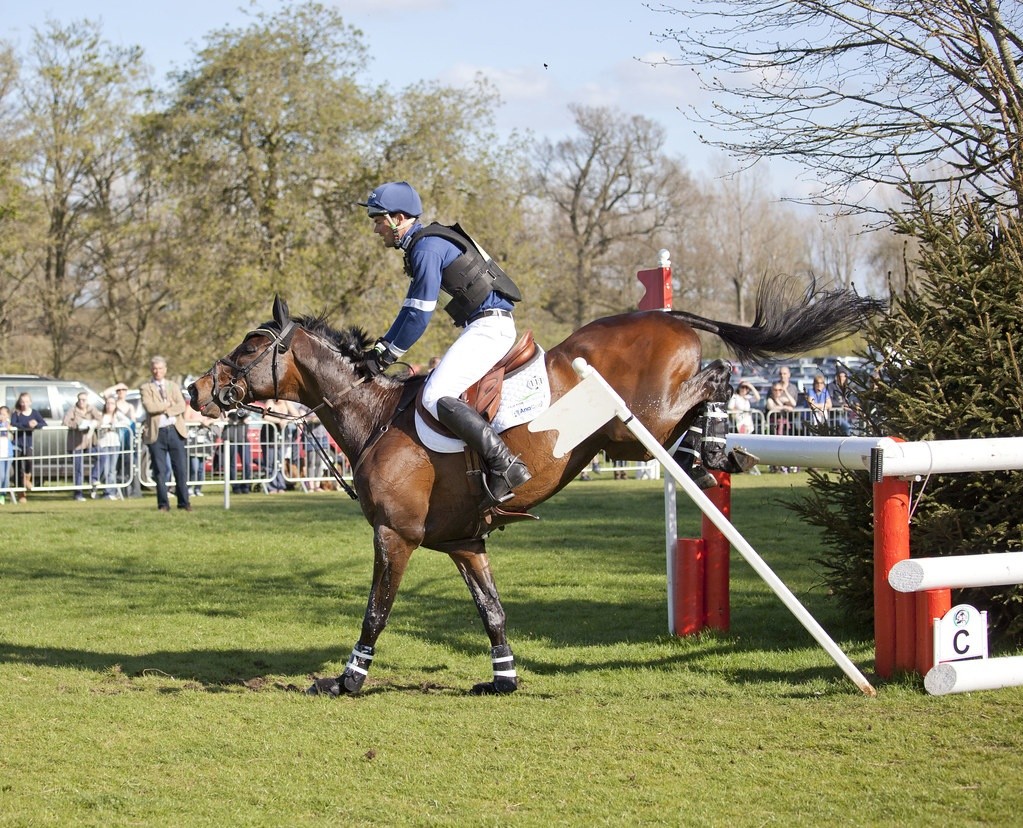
359,349,398,380
363,336,391,360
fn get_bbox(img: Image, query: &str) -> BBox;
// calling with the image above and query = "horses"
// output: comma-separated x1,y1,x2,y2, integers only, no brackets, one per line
184,270,893,695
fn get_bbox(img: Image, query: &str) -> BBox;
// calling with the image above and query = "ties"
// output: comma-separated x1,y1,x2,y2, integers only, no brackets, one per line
159,384,169,420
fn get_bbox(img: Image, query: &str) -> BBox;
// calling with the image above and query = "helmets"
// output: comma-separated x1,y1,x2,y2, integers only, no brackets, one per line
357,181,423,217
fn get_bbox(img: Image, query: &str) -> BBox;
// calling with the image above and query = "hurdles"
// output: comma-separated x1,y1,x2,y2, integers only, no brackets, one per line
636,245,1023,697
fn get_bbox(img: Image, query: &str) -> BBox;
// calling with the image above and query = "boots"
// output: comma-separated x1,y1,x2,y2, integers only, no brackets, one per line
437,396,532,514
23,472,33,489
17,491,27,503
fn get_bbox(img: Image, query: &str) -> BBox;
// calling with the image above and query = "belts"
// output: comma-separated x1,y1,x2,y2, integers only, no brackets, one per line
462,311,511,328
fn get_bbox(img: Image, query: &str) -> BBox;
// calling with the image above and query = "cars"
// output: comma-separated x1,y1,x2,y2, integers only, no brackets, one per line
105,379,356,472
697,350,902,437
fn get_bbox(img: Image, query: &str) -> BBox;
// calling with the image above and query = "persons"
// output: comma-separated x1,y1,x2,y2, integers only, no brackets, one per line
0,391,47,505
579,451,628,481
727,365,852,475
63,381,141,502
355,181,533,512
139,356,191,511
187,400,329,498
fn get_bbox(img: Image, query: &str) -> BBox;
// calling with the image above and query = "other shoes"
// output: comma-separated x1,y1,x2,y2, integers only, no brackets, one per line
748,464,819,474
314,487,324,492
195,489,204,497
178,505,191,511
593,465,601,474
308,488,314,493
0,494,5,506
105,494,118,501
615,474,620,479
621,474,628,479
580,475,592,481
160,506,169,512
74,495,87,502
90,491,99,499
189,489,196,499
233,487,250,495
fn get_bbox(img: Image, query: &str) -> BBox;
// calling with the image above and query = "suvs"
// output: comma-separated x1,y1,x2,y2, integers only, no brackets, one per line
0,373,134,485
